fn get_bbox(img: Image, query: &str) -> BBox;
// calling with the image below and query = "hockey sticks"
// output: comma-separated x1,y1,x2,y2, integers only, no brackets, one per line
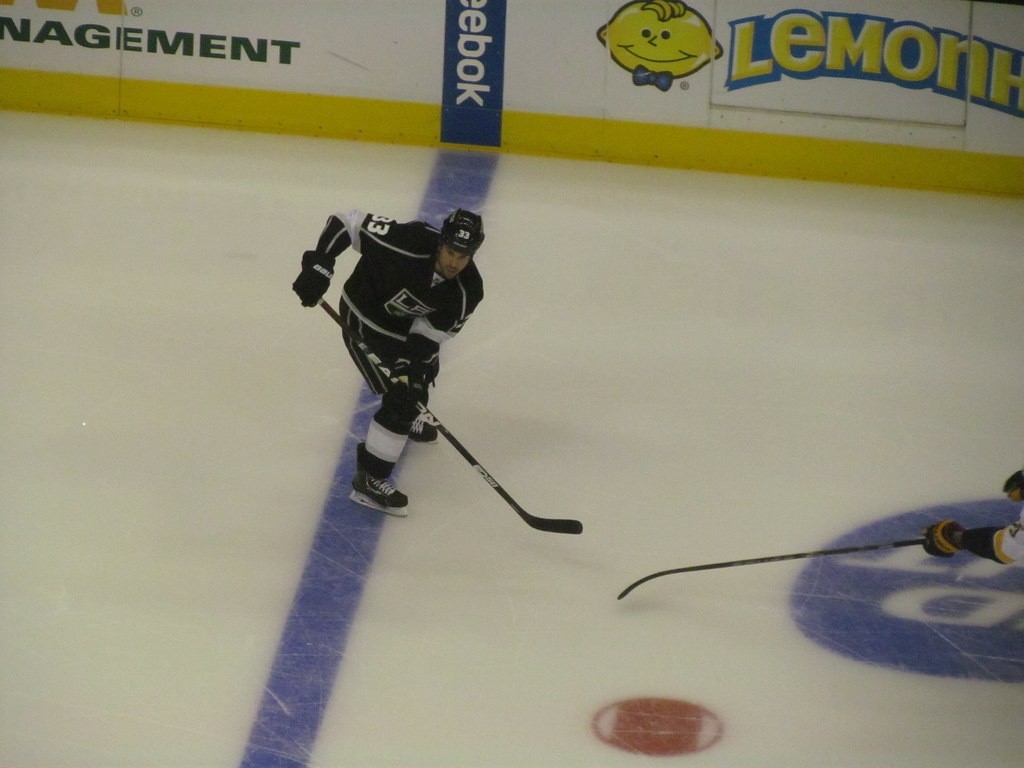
316,296,584,535
616,538,925,600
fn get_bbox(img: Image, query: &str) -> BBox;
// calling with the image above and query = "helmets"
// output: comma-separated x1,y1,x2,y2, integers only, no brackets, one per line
440,208,485,256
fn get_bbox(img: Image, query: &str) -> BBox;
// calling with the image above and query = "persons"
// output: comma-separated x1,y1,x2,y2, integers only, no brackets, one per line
291,208,486,517
921,469,1024,566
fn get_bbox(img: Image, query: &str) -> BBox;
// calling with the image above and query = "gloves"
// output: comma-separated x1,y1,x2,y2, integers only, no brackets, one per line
922,519,967,557
388,358,425,401
292,249,336,307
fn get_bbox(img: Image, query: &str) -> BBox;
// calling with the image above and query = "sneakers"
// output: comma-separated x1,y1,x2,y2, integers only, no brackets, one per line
351,459,408,518
409,420,440,444
